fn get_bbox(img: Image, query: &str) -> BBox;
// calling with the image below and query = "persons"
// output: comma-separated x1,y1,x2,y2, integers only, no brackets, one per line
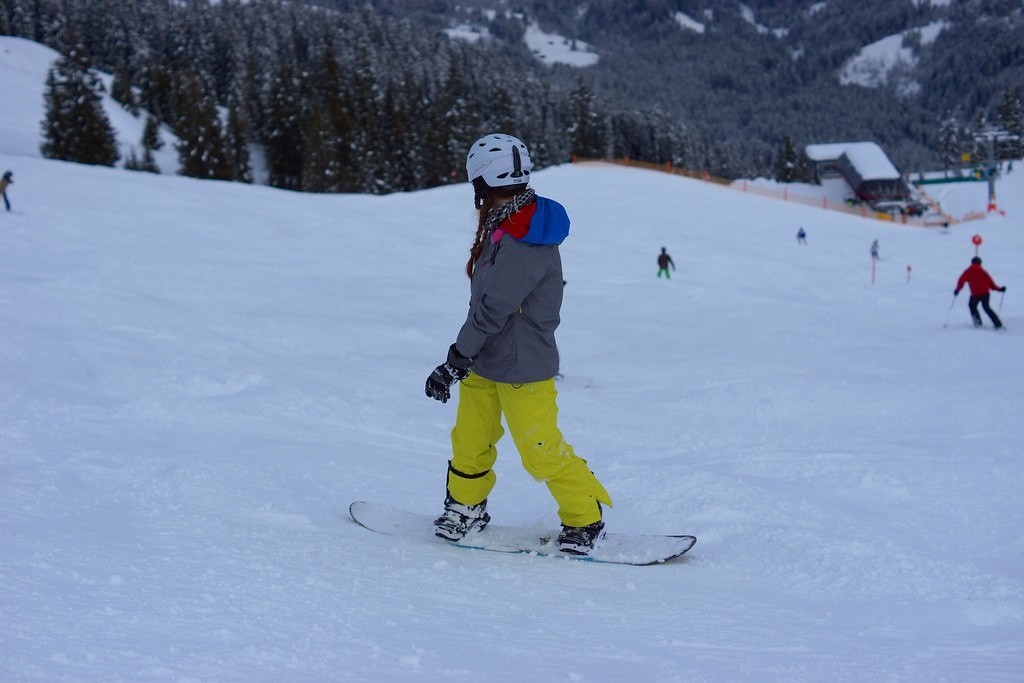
426,133,613,554
954,257,1006,329
0,170,14,210
944,221,948,229
871,240,880,258
657,247,675,280
797,228,806,244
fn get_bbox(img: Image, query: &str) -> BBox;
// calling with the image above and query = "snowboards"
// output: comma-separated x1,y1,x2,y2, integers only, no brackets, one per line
349,500,697,567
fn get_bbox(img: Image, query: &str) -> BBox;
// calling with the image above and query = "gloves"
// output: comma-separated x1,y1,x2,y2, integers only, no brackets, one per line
424,363,467,402
952,289,958,296
1000,285,1010,293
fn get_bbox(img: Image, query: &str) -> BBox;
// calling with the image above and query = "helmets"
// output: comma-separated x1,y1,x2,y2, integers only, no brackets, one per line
466,131,532,193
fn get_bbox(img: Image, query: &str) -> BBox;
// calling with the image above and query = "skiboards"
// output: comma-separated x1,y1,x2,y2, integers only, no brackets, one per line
963,323,1007,332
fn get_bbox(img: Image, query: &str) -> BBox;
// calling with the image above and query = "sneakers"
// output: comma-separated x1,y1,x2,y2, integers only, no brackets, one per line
557,520,607,555
433,498,491,540
